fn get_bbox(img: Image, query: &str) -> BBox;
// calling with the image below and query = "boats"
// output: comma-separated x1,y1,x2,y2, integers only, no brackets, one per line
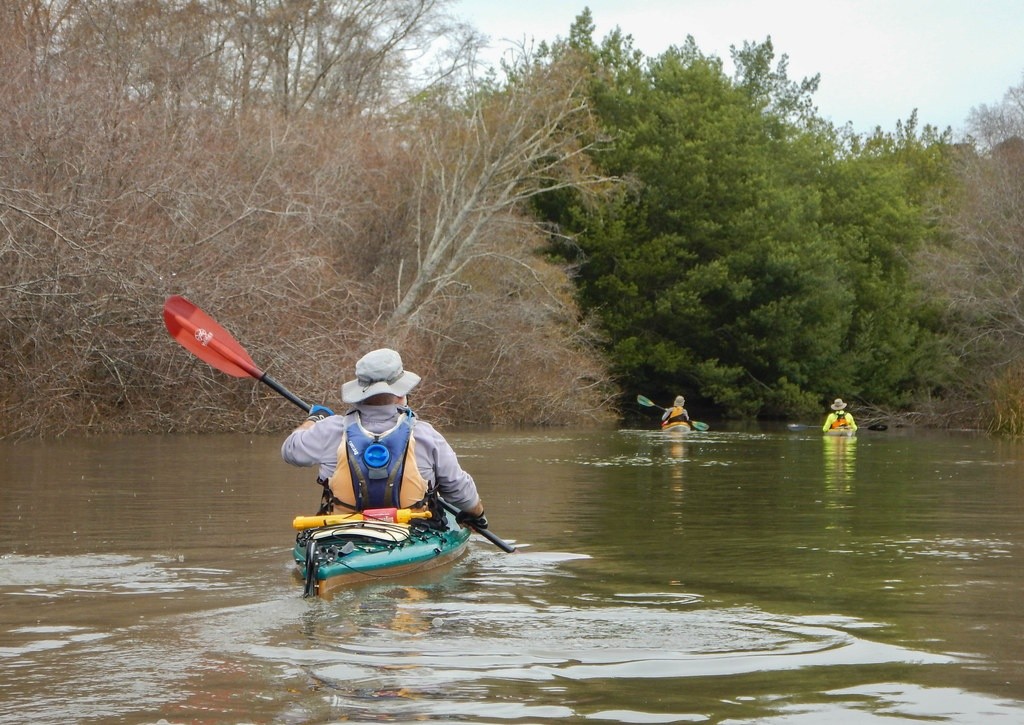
824,428,853,438
662,421,691,434
290,508,472,599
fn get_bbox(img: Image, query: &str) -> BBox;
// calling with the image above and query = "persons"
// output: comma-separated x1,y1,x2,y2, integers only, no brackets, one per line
662,396,692,423
280,347,488,531
822,397,859,432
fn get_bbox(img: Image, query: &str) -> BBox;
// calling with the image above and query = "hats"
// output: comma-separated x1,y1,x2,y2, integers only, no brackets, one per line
831,399,847,410
674,396,685,406
341,348,421,403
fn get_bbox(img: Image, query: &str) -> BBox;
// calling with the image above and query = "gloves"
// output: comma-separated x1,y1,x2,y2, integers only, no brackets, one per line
305,404,334,423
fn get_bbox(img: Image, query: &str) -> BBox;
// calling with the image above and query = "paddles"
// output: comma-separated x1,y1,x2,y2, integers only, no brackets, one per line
159,293,520,555
785,422,889,432
636,394,710,431
